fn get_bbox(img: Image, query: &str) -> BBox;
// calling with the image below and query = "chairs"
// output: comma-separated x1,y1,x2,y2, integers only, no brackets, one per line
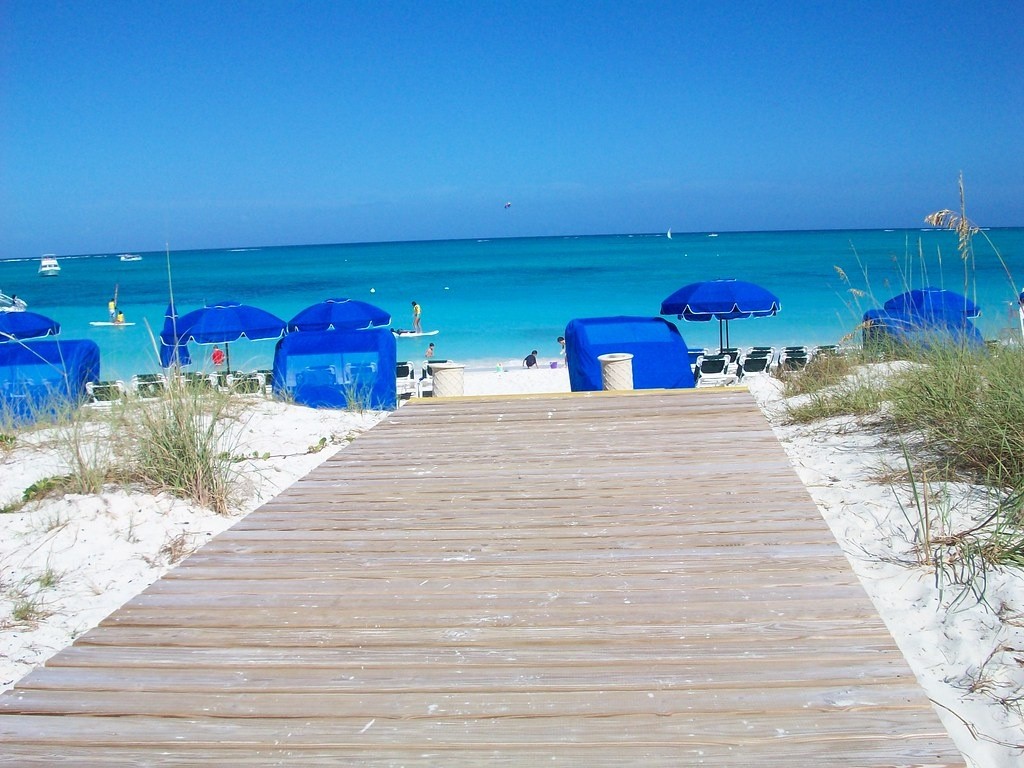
985,340,1000,356
688,345,838,388
81,359,453,401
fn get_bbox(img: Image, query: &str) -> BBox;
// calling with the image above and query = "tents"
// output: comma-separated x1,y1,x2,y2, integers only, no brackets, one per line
565,315,695,390
862,309,989,361
272,328,397,412
0,339,100,429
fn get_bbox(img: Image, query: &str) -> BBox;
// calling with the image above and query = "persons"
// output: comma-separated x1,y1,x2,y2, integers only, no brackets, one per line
212,345,225,373
523,350,539,369
109,298,116,322
557,337,567,366
114,311,125,325
425,343,434,357
412,301,422,333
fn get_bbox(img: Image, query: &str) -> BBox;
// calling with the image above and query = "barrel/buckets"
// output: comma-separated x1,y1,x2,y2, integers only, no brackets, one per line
550,361,557,369
496,363,502,372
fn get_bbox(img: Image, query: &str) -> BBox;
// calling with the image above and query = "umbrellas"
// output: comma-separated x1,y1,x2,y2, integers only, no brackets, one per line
287,298,391,383
660,278,782,354
0,311,61,344
158,302,193,369
884,287,981,319
160,301,287,373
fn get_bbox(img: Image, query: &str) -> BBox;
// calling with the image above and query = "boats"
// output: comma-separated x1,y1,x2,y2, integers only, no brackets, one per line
120,254,143,262
0,289,27,313
38,253,61,277
